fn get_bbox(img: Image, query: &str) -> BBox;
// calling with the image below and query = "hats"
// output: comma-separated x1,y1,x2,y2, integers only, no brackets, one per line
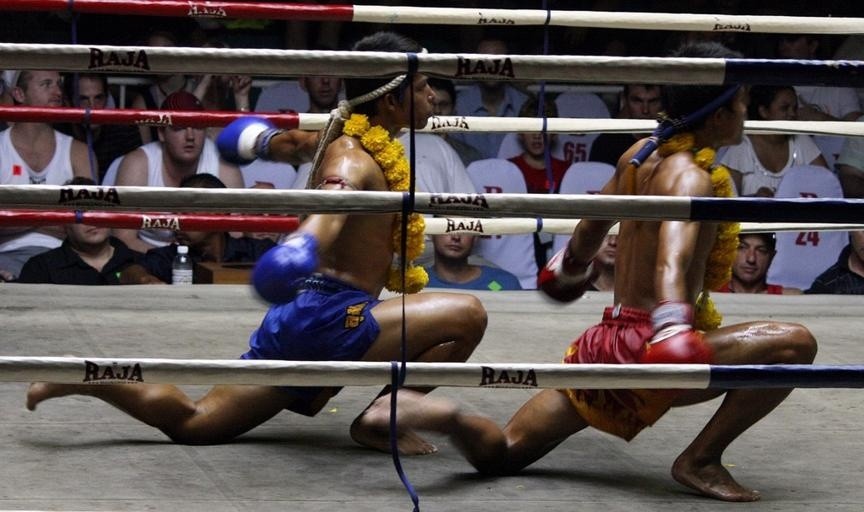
160,89,210,132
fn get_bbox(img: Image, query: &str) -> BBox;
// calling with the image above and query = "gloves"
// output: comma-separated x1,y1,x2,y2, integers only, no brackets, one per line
644,301,713,362
217,116,283,164
537,245,601,302
250,234,321,303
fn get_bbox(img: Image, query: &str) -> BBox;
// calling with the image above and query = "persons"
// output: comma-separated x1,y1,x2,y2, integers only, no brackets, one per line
363,45,819,500
0,33,864,295
26,32,488,456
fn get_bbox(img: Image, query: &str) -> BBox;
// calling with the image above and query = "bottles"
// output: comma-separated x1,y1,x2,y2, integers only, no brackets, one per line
171,245,195,283
